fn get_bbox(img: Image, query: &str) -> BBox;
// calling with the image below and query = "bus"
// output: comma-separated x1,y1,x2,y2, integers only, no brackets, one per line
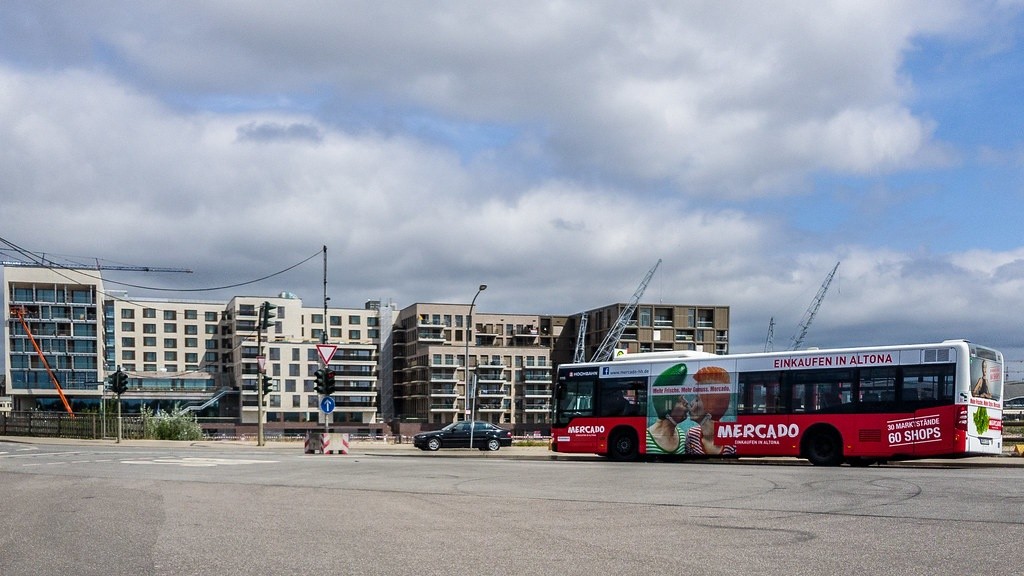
547,337,1005,470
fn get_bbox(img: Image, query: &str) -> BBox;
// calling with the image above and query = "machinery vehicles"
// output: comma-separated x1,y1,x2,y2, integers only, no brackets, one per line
764,262,841,350
571,257,662,363
10,304,77,419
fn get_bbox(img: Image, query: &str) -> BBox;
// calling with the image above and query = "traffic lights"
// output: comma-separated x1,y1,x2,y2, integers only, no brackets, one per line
314,369,324,394
264,375,273,395
108,373,117,392
263,300,277,330
326,369,335,394
118,372,128,394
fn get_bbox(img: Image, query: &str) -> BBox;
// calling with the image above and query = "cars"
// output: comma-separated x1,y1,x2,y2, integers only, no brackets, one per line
413,420,512,451
1003,396,1024,408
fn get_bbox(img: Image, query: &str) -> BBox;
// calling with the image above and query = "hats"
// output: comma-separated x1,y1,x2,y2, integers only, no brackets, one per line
652,363,687,420
693,366,730,421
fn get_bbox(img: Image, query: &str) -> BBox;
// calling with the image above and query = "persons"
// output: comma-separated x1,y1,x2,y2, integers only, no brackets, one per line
972,360,992,398
646,363,737,455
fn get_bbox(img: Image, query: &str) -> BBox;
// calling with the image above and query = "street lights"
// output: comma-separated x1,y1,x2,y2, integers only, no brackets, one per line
464,283,489,420
